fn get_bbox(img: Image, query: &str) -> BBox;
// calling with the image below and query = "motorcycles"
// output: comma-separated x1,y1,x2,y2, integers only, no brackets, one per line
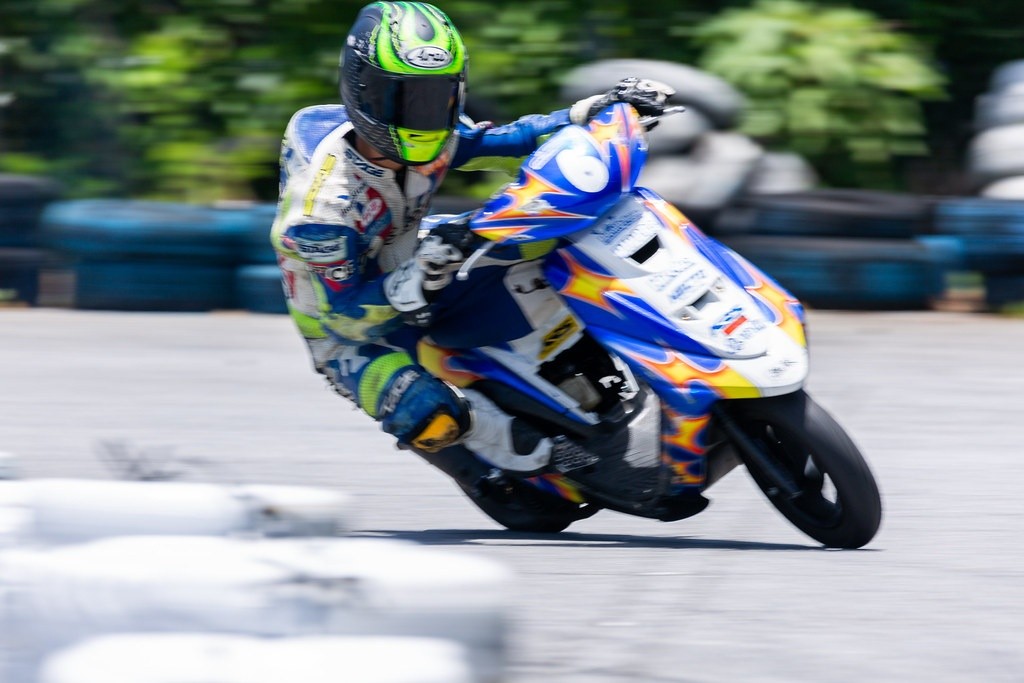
415,95,882,551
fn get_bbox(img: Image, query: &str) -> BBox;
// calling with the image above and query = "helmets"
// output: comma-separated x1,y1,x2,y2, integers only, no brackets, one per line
339,1,469,166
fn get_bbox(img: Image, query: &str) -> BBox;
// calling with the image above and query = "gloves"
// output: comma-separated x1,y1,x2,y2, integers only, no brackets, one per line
389,223,479,326
570,77,676,130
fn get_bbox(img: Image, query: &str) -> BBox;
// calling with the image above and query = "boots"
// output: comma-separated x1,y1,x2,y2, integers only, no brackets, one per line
457,387,553,474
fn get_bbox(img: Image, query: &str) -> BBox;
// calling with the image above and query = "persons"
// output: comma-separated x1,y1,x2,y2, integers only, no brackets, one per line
268,0,677,474
